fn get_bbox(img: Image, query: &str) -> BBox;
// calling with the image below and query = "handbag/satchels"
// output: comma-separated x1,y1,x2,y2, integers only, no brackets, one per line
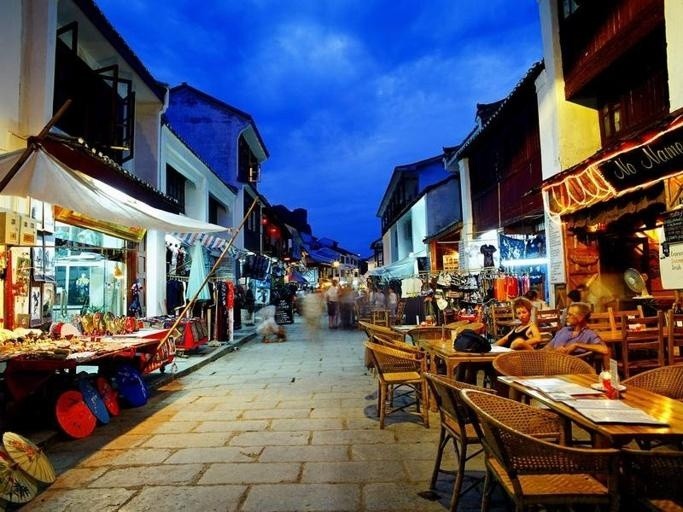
454,329,491,352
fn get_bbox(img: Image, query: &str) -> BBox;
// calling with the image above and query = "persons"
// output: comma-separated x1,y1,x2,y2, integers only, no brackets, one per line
510,302,609,356
456,297,541,384
525,287,592,327
254,298,284,343
324,280,398,329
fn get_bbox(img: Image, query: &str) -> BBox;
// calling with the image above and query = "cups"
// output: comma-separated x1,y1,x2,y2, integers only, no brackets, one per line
426,316,433,326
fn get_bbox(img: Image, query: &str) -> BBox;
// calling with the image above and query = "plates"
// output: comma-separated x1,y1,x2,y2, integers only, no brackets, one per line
82,312,135,335
590,383,627,392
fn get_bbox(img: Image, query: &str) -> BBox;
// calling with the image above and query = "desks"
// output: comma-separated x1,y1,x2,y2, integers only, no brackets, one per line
8,311,209,428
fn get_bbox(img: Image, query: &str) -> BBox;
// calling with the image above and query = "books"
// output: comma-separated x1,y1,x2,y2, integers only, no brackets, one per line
514,378,669,426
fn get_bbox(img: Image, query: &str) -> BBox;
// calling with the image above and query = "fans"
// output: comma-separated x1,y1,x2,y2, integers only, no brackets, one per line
623,266,654,299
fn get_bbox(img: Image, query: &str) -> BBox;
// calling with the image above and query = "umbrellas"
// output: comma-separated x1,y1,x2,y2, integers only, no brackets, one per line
1,365,149,505
